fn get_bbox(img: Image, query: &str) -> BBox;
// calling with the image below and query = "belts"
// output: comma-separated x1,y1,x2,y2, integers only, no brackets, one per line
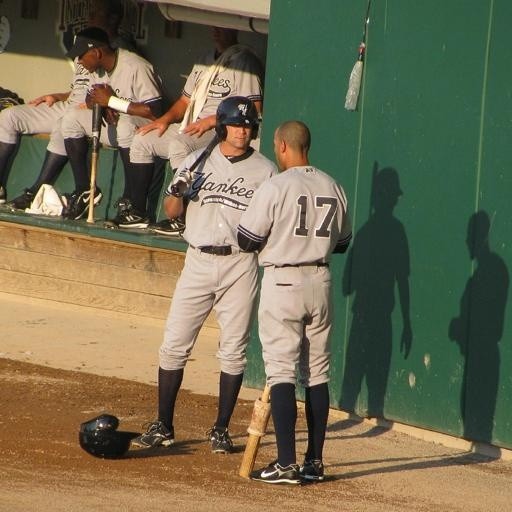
274,262,329,268
199,245,231,257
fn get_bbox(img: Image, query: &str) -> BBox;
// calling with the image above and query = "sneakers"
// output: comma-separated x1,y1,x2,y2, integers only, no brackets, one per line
151,217,187,237
250,460,301,486
206,426,232,454
113,211,149,230
65,187,103,219
4,185,33,210
298,457,326,482
129,422,177,447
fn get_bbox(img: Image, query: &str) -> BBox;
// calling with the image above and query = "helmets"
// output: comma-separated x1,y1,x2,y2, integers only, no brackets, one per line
213,95,263,130
78,413,144,459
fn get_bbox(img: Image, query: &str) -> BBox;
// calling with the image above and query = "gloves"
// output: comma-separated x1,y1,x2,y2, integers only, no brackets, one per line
170,169,195,196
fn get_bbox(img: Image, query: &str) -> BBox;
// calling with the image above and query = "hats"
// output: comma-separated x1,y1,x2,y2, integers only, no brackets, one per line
66,25,112,57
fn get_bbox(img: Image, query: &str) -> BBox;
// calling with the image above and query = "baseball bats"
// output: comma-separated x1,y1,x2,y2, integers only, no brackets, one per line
171,133,219,199
85,101,102,226
238,377,272,478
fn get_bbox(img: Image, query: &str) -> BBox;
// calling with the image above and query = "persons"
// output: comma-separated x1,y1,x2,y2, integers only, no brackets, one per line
236,120,353,485
105,22,265,237
61,27,164,228
0,0,133,223
129,95,282,453
